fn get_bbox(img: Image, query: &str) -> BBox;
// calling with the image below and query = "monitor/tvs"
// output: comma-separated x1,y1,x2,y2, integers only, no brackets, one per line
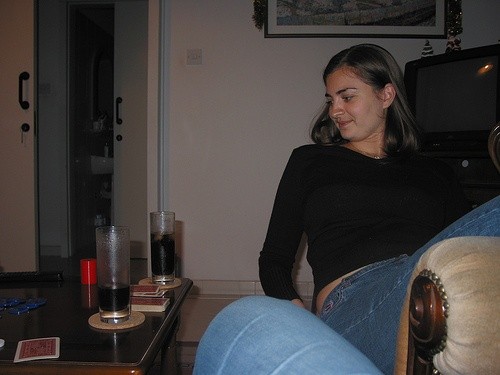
403,43,500,151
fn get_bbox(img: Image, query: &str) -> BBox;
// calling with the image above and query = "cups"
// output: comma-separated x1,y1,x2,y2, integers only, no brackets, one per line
80,259,97,284
150,211,175,285
96,226,131,323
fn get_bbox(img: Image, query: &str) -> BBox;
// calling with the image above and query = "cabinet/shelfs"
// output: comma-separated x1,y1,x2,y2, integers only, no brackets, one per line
420,151,500,201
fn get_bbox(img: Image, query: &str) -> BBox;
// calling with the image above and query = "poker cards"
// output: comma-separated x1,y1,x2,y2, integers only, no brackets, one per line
14,336,60,364
129,284,170,312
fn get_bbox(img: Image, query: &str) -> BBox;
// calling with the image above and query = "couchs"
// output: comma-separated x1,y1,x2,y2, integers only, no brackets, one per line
193,236,500,375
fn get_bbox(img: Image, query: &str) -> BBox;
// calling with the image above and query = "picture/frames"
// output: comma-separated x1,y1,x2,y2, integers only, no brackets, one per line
264,0,448,39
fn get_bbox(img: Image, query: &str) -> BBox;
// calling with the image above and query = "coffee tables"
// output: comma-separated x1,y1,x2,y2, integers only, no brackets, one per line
0,272,193,374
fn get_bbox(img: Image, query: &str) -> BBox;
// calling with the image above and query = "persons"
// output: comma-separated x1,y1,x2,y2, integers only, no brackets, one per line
258,42,500,375
191,294,385,375
